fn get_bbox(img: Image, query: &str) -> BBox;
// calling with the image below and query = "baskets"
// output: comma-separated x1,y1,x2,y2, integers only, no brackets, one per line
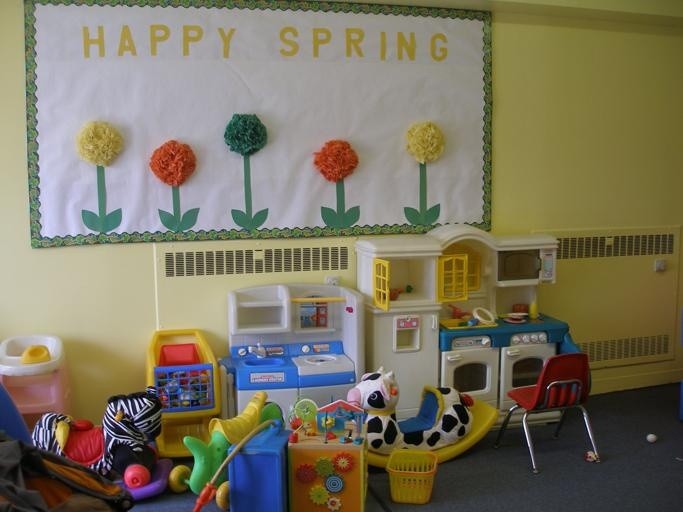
385,445,438,504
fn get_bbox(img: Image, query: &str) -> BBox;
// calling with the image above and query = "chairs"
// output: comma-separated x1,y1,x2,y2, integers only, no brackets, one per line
494,353,601,475
